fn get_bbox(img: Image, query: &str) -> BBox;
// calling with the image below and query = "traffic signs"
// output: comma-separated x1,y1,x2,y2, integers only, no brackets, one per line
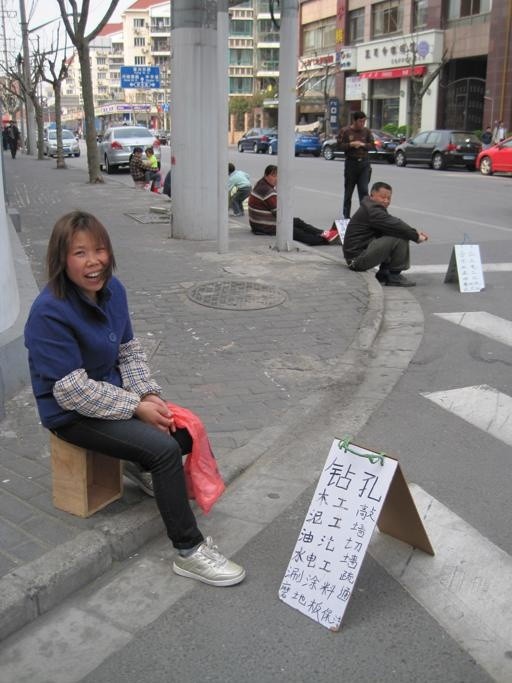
163,104,169,112
121,66,161,90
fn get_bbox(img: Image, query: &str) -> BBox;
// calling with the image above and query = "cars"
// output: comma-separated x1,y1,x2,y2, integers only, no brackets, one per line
392,129,482,170
237,127,276,153
40,128,81,157
320,128,402,163
96,125,163,174
267,130,320,156
152,130,167,144
476,134,512,175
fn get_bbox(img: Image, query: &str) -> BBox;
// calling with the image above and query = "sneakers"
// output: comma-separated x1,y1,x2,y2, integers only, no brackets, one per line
173,544,246,587
326,230,339,242
123,462,154,497
375,271,416,287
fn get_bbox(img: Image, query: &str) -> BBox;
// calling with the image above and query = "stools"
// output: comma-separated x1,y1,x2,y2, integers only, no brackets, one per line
134,180,146,191
48,435,127,518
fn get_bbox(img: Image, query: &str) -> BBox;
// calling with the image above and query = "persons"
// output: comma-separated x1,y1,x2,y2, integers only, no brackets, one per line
129,148,162,194
163,170,171,198
24,211,246,587
337,111,374,218
144,146,158,191
228,163,252,217
5,121,20,159
343,182,428,286
481,126,493,150
248,164,338,246
2,126,8,151
492,120,508,146
